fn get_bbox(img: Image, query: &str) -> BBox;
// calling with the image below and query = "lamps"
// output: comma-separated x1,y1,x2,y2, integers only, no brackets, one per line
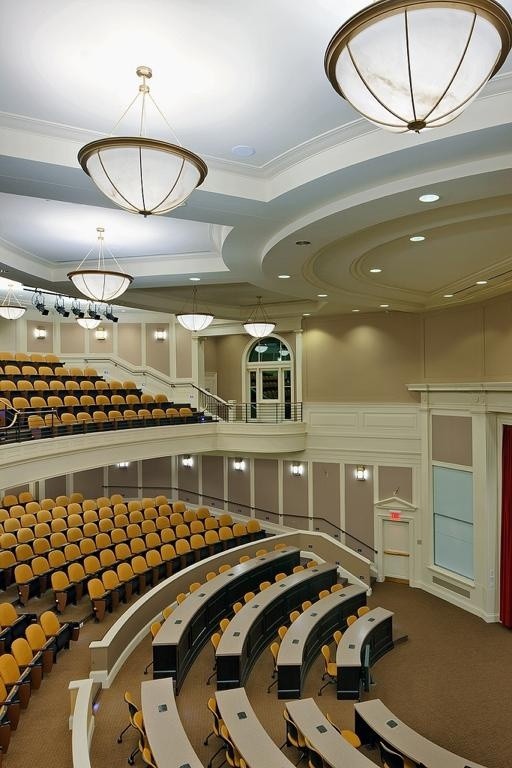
0,284,27,320
76,305,103,330
117,462,129,468
156,327,166,341
23,288,119,322
96,326,106,340
175,286,215,331
182,454,192,470
37,326,46,339
292,460,301,476
279,350,289,356
77,66,208,216
234,457,243,472
66,227,134,303
255,343,268,353
323,0,512,136
241,296,277,338
356,464,366,481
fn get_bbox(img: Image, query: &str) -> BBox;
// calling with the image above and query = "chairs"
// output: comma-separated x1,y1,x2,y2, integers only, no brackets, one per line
0,352,219,439
